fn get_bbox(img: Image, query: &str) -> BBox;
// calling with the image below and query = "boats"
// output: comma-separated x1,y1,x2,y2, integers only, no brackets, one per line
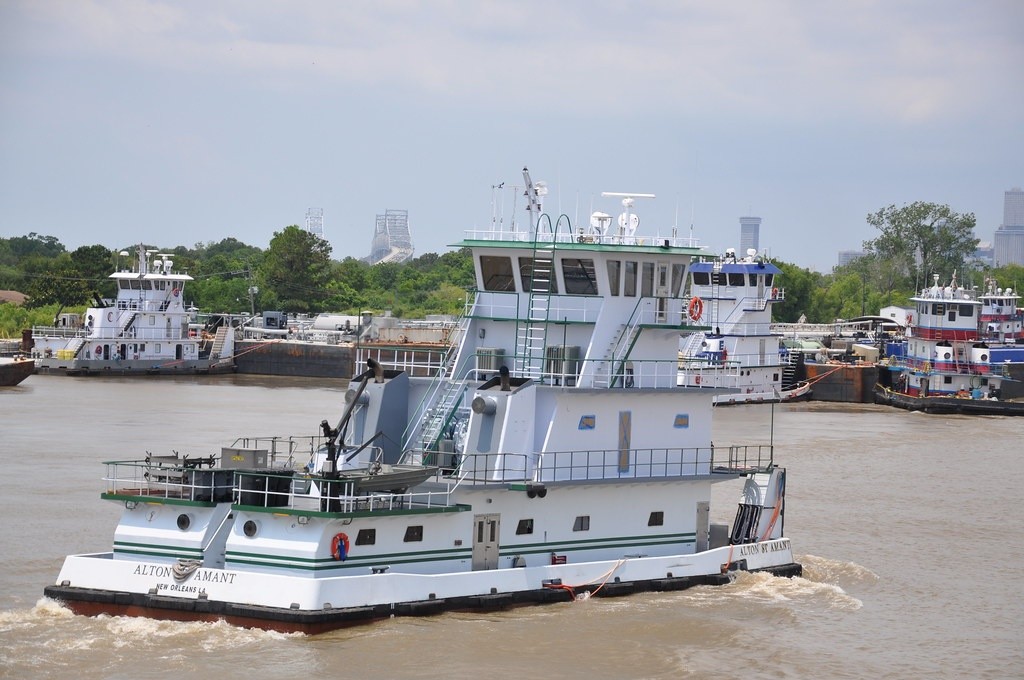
676,247,813,406
0,355,35,387
872,269,1024,417
28,243,240,376
42,166,804,634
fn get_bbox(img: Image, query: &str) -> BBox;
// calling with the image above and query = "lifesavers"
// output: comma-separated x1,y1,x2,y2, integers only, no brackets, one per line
906,314,913,324
173,288,180,297
773,288,778,296
332,532,350,561
695,373,701,385
96,346,102,354
689,296,703,321
